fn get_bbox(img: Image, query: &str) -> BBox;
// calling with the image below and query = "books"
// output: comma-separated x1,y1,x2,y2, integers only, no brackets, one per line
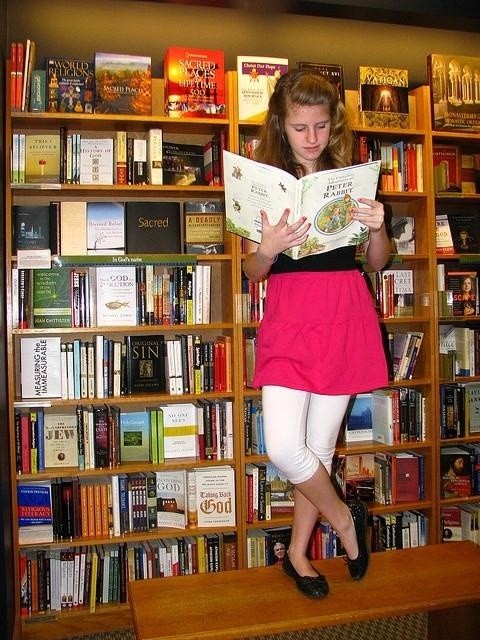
427,54,480,134
367,214,480,319
237,51,357,125
346,326,480,557
7,39,226,119
12,200,232,401
236,244,346,568
360,135,480,194
15,398,236,620
221,149,383,263
13,127,227,186
358,66,410,129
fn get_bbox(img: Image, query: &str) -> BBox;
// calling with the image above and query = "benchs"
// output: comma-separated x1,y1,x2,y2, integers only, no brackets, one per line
124,539,480,638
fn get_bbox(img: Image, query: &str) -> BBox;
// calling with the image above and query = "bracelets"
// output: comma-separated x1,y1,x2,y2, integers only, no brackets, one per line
254,244,279,265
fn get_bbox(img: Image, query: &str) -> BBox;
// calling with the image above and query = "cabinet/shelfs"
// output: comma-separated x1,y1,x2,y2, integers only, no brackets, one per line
427,85,479,547
234,70,436,572
4,70,241,639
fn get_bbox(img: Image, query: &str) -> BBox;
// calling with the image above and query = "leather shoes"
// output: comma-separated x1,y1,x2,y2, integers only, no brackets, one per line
345,498,371,583
281,548,329,601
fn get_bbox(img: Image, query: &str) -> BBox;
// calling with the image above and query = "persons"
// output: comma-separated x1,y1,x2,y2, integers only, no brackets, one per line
252,61,391,602
